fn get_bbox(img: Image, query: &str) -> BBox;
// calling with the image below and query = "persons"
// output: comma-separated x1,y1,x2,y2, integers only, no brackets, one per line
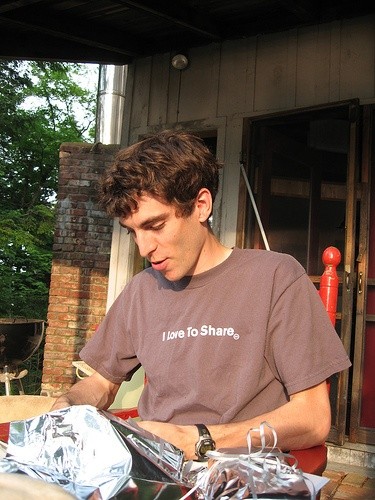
49,128,352,462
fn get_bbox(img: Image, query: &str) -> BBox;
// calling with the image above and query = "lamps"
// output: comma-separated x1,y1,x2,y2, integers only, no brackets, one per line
170,50,191,71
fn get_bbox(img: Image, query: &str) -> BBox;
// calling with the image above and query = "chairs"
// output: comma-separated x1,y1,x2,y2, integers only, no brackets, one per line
0,247,340,500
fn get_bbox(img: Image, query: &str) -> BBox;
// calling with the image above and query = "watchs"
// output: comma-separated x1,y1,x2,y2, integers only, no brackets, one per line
194,423,217,461
